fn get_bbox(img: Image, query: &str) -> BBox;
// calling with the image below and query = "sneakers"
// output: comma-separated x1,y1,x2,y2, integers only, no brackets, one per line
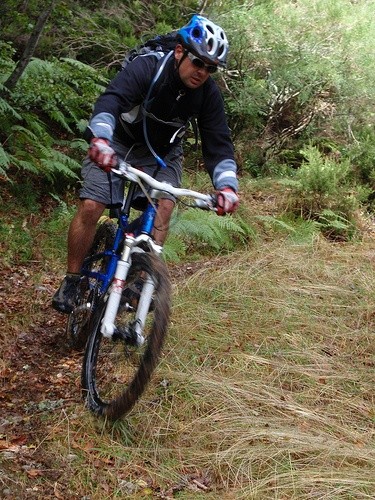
52,278,84,313
123,284,155,314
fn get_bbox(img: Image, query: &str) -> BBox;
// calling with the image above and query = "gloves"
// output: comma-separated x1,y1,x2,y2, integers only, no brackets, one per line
213,187,240,216
88,138,117,172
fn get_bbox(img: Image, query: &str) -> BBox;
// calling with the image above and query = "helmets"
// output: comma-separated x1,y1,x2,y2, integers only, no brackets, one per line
175,14,228,66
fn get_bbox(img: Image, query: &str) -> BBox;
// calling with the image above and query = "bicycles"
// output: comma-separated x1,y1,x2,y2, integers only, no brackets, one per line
63,127,218,421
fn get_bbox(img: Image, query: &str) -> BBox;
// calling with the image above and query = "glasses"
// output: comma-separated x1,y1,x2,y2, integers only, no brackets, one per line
181,47,218,74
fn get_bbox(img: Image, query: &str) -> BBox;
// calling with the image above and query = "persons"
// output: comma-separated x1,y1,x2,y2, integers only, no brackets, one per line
50,13,241,312
